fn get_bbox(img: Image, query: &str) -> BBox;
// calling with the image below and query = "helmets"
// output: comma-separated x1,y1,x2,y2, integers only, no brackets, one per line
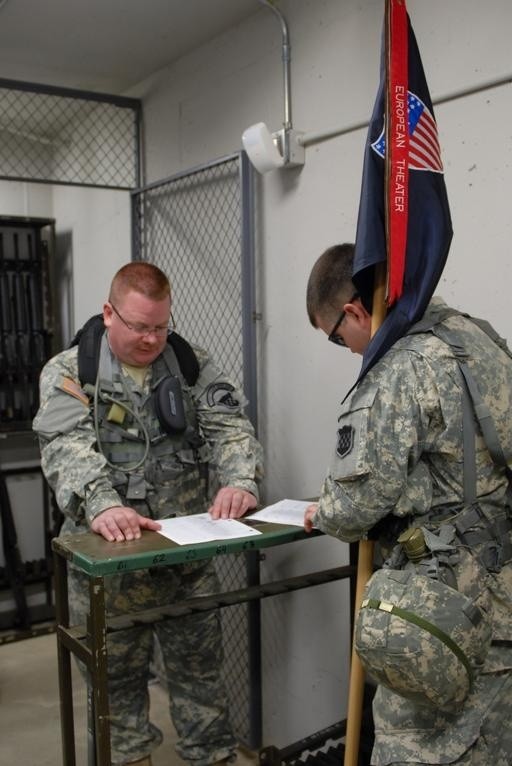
352,568,494,718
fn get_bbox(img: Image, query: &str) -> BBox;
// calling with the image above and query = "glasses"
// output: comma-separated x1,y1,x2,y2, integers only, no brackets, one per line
328,292,361,348
108,300,176,337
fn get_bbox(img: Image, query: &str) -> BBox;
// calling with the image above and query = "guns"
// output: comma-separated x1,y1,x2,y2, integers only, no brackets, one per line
1,272,44,424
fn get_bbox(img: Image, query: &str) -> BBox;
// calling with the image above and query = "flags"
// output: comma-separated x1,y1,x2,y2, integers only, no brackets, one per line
341,0,454,408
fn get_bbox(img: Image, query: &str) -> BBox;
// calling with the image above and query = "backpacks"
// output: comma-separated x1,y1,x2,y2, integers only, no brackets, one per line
70,314,200,387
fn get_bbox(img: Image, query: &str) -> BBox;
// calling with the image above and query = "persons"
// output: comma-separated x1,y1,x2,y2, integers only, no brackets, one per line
31,261,265,766
303,244,512,766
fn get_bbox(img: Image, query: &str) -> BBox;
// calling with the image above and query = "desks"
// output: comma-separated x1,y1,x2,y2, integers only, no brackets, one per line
50,495,382,765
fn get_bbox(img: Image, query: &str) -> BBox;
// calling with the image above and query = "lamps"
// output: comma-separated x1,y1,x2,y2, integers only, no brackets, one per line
241,122,305,175
